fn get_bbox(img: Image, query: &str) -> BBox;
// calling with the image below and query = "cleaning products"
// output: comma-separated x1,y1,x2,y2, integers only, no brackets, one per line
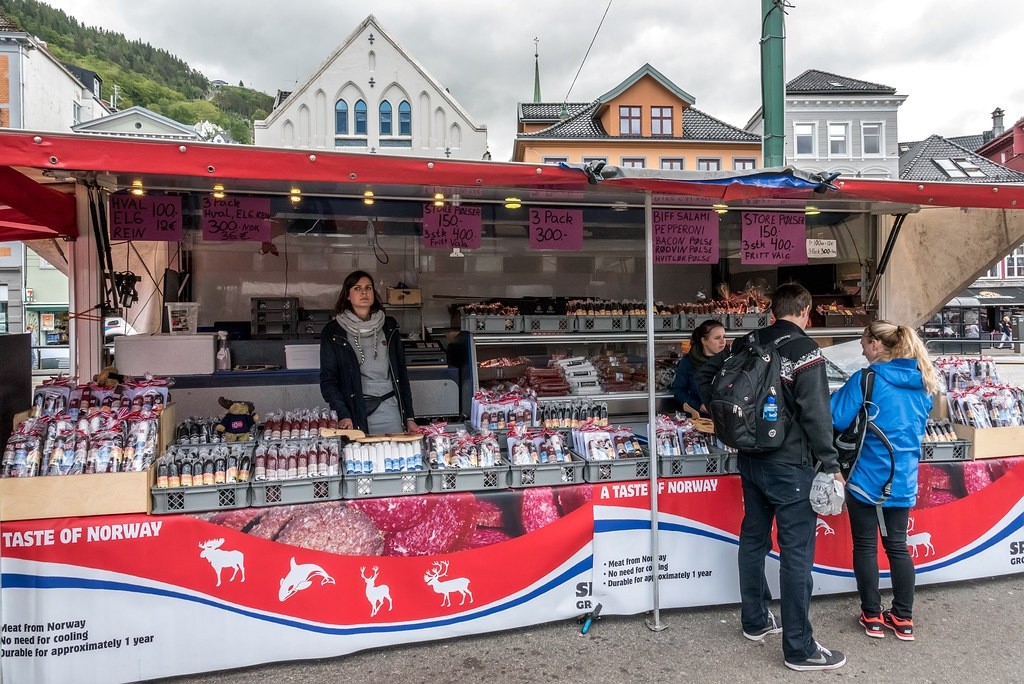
216,329,232,372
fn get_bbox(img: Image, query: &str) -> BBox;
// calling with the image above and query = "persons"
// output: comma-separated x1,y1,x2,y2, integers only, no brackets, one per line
319,271,419,435
670,317,733,415
969,319,980,336
830,317,947,643
996,316,1015,350
727,280,850,671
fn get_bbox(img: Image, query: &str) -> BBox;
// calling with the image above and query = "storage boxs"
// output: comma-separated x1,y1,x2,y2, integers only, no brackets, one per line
459,311,774,335
164,302,200,337
283,344,320,369
815,305,872,327
385,285,423,305
114,331,217,376
147,412,975,516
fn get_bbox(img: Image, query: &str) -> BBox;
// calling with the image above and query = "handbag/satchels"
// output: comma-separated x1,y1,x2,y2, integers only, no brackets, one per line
363,389,396,417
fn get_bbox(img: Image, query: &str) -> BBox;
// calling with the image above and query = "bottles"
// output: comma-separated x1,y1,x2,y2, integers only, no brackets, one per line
217,331,231,372
761,396,777,422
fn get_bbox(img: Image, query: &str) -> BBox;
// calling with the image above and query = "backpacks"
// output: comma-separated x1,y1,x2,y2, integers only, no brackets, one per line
813,367,895,504
710,329,813,454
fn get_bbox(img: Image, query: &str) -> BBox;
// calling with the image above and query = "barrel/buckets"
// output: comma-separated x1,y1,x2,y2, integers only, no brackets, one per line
164,300,200,336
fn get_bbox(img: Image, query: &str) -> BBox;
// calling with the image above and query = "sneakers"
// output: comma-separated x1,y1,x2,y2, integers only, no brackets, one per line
881,609,915,640
742,615,783,641
858,611,885,638
784,647,847,671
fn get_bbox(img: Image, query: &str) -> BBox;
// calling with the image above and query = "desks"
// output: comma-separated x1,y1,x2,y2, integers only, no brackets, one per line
0,455,1022,684
125,362,462,430
